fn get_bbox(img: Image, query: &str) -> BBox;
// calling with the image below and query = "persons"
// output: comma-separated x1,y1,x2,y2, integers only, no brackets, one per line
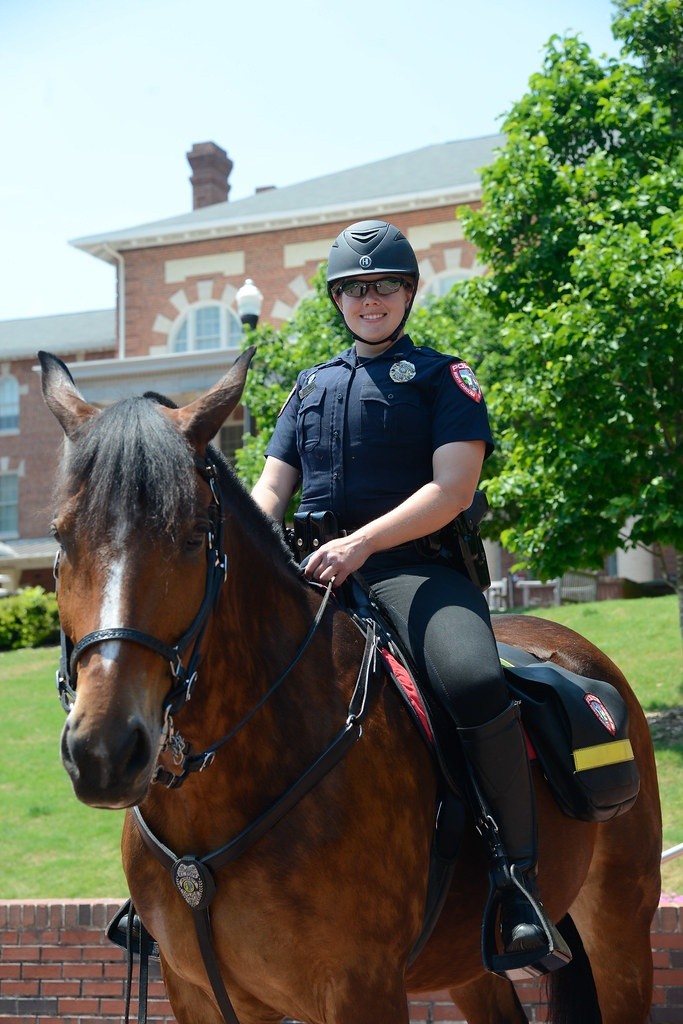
118,219,557,971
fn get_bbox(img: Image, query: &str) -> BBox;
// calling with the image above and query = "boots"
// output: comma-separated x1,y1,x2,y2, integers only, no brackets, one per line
456,699,549,954
118,913,150,939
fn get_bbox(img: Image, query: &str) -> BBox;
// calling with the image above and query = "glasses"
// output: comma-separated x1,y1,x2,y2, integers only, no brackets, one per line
336,277,412,296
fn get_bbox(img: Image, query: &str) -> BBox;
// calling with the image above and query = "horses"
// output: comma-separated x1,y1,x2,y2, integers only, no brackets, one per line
36,350,662,1024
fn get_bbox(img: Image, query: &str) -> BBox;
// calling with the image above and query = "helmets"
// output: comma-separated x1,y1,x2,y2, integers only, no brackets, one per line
325,220,419,283
